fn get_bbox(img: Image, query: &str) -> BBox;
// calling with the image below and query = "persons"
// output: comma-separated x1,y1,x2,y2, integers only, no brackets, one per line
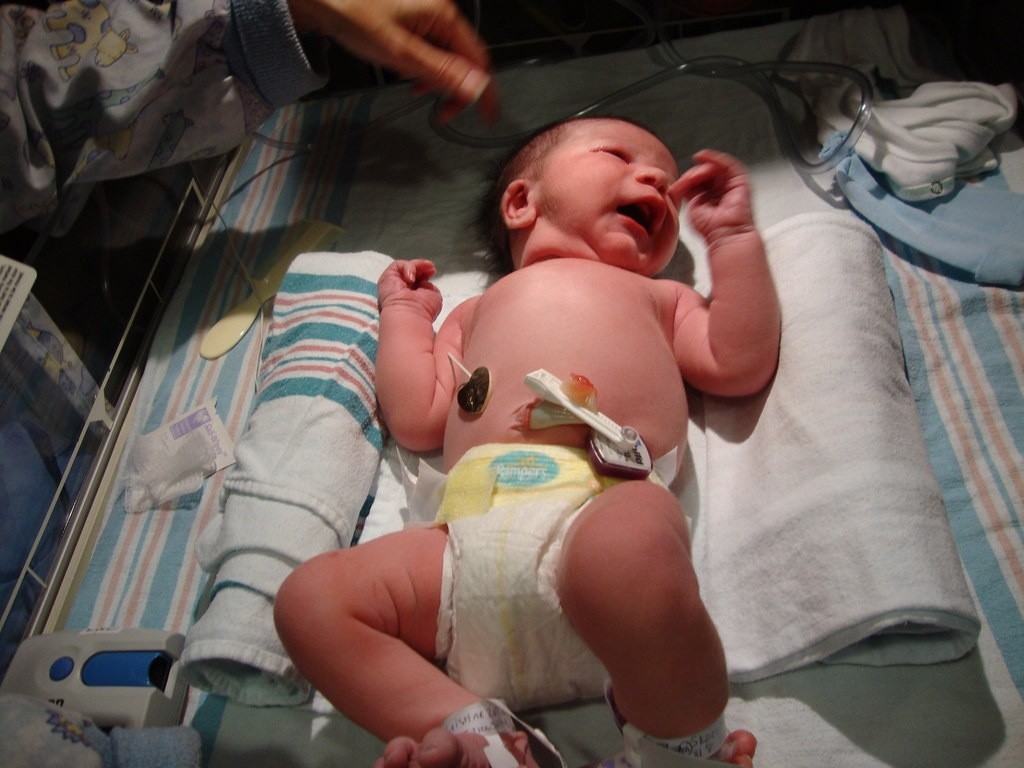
0,0,501,194
276,117,780,768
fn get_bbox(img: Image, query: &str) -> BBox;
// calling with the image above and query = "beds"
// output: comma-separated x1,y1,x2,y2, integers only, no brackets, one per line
0,0,1024,768
